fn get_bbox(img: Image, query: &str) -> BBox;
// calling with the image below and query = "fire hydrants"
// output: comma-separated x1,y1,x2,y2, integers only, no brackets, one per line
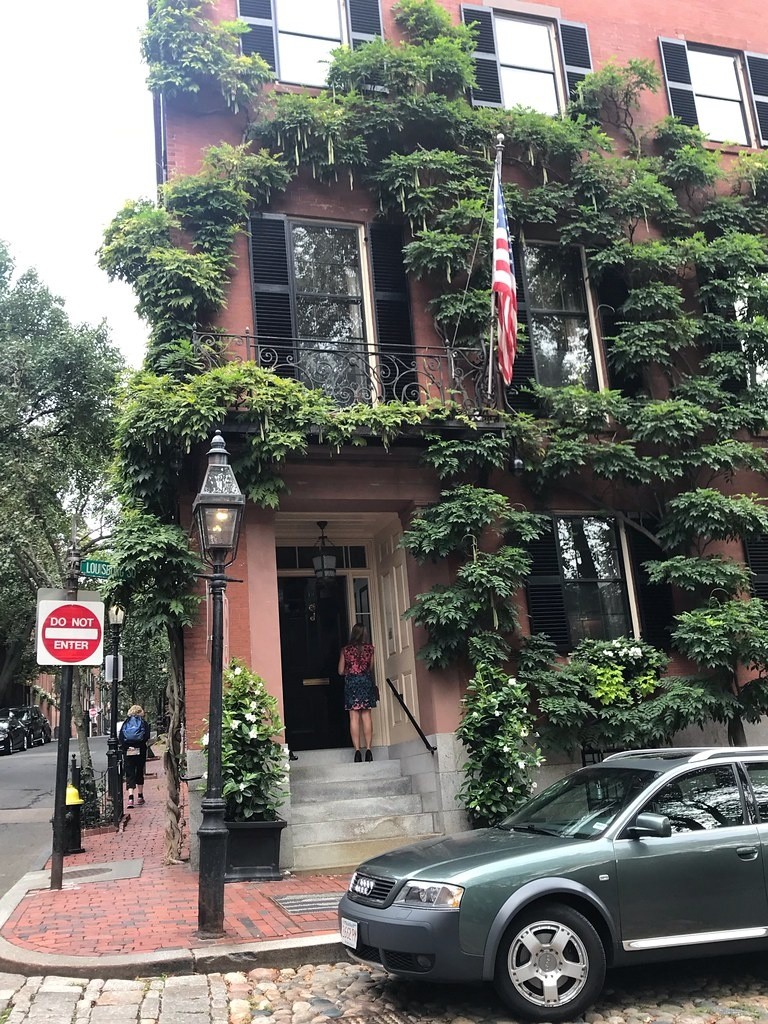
64,783,85,854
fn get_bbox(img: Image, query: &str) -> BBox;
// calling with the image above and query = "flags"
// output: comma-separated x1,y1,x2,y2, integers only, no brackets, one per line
491,162,517,387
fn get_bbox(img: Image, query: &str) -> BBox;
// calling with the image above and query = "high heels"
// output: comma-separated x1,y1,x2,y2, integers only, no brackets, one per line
355,750,362,762
366,750,372,762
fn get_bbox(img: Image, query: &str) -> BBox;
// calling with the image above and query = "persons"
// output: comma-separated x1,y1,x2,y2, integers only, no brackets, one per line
338,624,380,763
119,705,150,808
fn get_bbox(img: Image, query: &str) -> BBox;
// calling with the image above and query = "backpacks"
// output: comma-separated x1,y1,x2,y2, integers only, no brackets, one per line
122,715,146,742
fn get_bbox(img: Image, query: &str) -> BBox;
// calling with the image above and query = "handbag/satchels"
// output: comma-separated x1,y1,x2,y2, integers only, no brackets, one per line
375,686,380,701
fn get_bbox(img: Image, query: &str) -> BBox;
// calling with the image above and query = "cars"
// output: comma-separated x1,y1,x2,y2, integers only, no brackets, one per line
0,706,52,756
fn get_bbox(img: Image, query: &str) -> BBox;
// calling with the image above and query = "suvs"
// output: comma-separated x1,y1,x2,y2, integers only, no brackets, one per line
337,745,768,1022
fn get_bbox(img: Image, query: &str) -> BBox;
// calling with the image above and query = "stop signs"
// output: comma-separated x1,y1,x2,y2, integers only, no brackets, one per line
34,588,104,668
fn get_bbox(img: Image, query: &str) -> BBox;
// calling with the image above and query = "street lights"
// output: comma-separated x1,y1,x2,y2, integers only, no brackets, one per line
183,425,247,940
109,592,129,822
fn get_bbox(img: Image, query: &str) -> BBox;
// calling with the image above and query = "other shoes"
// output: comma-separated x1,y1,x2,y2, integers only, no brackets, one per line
127,800,135,808
137,797,145,805
